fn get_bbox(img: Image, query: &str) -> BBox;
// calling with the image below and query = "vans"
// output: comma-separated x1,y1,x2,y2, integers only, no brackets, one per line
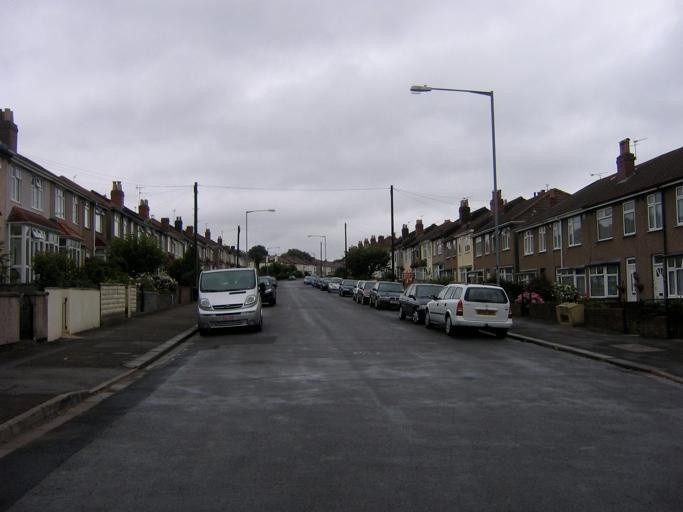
197,267,263,337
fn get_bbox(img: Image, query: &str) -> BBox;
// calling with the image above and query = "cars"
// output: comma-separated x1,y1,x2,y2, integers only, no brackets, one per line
424,284,513,339
399,284,444,324
353,280,404,309
258,276,278,306
304,276,358,296
289,276,296,280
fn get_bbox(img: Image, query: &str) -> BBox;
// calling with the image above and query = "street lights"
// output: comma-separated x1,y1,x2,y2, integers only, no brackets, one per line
306,235,326,277
246,210,275,266
411,85,500,286
